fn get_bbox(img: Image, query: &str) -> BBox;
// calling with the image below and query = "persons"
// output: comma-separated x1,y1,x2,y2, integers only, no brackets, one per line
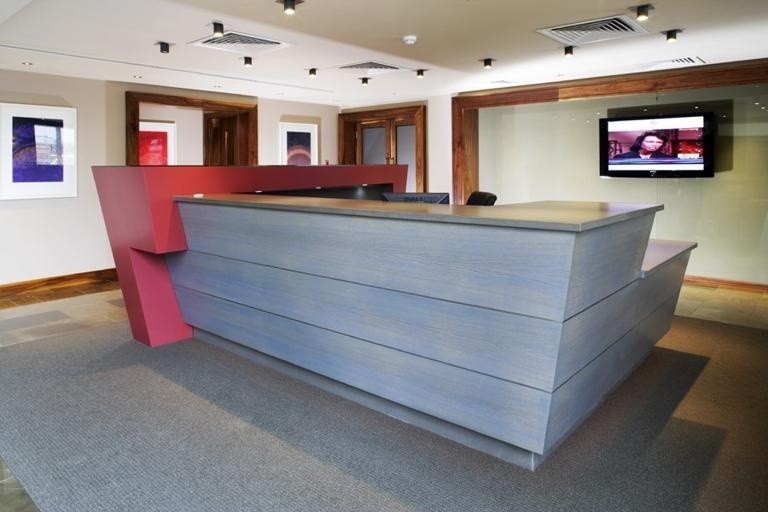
613,129,677,160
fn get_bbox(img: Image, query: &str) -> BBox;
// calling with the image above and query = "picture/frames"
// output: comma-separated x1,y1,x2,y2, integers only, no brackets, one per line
0,101,78,201
140,119,176,166
281,123,321,165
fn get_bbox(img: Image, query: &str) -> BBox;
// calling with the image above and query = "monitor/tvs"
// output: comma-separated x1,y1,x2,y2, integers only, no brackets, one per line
599,112,714,178
381,192,449,204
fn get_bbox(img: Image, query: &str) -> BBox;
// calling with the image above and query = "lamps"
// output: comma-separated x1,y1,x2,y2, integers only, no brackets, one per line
362,78,368,83
310,69,316,76
483,59,492,67
666,30,675,41
161,44,170,54
565,46,573,55
213,24,223,37
284,2,296,15
637,5,649,21
244,57,252,65
418,70,424,76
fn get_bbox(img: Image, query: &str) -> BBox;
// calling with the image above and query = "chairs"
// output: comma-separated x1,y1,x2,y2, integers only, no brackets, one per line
467,190,496,206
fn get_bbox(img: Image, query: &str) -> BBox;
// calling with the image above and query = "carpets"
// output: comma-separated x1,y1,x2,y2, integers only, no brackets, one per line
1,318,767,512
